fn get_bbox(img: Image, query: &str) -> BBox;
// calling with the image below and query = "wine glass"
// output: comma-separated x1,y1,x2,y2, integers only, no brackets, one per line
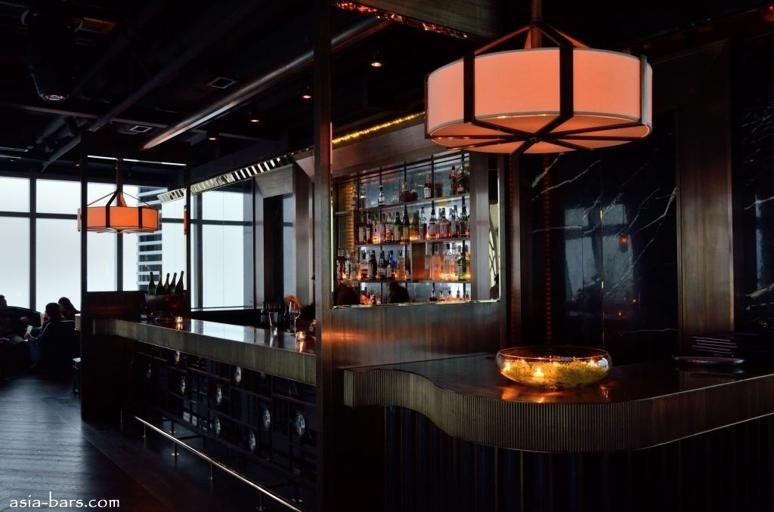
289,302,301,333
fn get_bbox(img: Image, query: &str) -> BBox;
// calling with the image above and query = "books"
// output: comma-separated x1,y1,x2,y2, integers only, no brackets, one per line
672,331,750,369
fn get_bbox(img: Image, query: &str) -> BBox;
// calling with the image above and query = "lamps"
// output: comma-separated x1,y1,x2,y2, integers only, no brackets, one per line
422,0,654,157
77,152,159,233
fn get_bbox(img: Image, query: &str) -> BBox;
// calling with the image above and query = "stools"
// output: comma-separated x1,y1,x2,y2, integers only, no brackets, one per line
72,358,81,398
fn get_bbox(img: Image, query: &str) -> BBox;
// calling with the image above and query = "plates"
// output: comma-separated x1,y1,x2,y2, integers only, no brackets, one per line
666,329,759,367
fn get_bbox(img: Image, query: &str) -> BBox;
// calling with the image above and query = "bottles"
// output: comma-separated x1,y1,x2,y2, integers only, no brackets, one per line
260,301,288,327
430,288,470,301
356,197,474,244
357,158,473,209
148,271,187,294
336,239,473,281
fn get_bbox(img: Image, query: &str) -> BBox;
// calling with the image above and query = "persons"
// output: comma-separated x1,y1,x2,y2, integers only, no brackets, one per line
56,297,80,321
24,303,59,341
0,295,31,346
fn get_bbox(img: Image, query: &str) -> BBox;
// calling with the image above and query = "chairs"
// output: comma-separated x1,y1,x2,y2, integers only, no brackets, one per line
30,320,75,380
0,306,41,376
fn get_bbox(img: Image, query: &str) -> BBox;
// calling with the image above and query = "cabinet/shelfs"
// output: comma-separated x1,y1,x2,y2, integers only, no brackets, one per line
333,145,472,302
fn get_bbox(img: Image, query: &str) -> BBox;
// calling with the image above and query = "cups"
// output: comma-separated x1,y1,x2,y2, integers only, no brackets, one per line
268,312,288,339
